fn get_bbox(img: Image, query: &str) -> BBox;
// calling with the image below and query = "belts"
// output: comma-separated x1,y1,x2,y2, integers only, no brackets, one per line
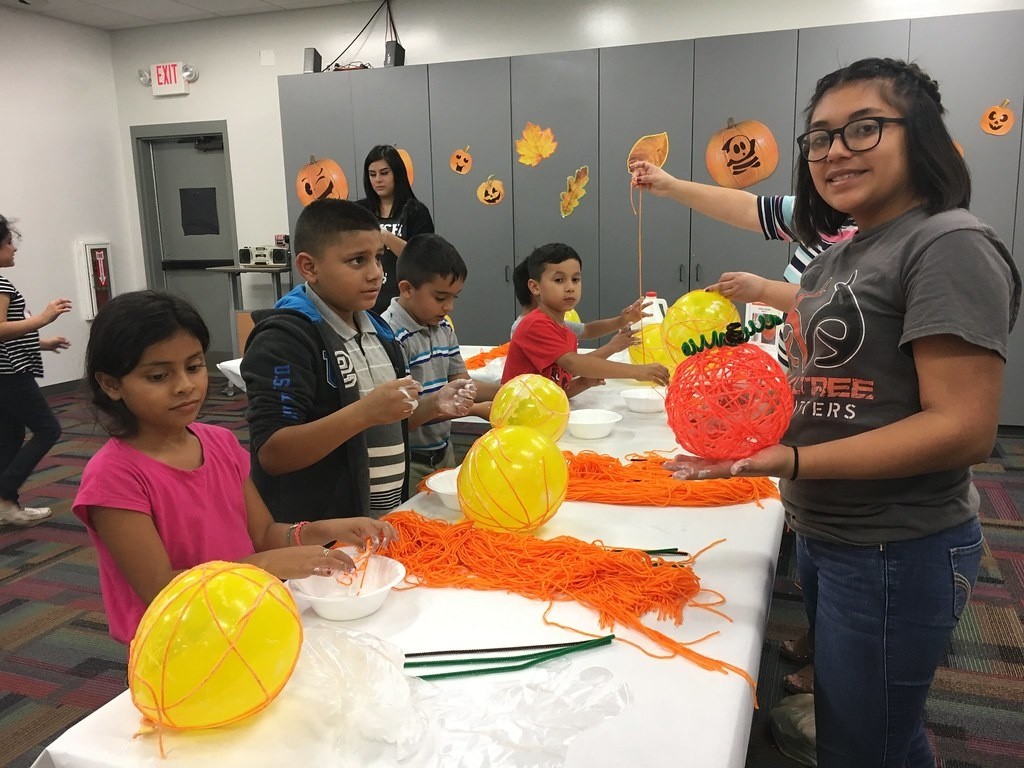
411,448,445,469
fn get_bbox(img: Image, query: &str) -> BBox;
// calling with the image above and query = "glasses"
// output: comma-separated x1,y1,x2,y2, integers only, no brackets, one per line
796,117,904,162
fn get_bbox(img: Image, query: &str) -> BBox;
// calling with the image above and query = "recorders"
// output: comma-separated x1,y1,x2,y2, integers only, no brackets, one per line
238,245,289,266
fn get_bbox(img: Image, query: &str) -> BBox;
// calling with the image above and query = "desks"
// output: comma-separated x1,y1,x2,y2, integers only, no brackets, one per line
35,347,790,768
206,265,291,397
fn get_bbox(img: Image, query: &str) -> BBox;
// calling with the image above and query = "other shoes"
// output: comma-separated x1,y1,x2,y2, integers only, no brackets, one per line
0,500,51,525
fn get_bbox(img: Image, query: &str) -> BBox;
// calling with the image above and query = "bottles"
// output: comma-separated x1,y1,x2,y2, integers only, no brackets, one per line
631,292,669,330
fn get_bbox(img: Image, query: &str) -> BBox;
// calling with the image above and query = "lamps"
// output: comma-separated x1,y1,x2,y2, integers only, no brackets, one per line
136,62,198,97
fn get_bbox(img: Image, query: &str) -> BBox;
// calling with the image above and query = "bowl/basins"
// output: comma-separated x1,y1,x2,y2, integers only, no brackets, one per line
286,552,406,621
620,388,666,414
566,409,623,439
425,470,462,511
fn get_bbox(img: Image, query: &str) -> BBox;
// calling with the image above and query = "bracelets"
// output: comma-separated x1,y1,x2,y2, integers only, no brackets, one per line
295,520,310,545
790,446,798,483
287,523,299,547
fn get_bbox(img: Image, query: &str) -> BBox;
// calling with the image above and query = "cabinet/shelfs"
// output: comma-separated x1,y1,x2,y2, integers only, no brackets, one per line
276,11,1024,428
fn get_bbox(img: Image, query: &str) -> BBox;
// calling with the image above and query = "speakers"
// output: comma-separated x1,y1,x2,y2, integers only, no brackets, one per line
384,41,406,67
302,47,322,74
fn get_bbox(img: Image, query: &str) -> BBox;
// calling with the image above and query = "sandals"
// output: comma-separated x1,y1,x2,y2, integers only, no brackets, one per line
780,629,814,662
782,663,815,694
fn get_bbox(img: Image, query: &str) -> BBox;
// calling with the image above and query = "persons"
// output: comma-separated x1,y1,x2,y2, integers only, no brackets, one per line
663,56,1022,768
629,158,861,696
500,242,671,397
509,257,653,360
73,290,397,649
379,233,503,498
0,214,72,527
240,200,479,547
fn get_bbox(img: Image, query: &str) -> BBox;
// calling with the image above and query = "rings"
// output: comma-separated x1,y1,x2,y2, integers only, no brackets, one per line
323,548,329,557
